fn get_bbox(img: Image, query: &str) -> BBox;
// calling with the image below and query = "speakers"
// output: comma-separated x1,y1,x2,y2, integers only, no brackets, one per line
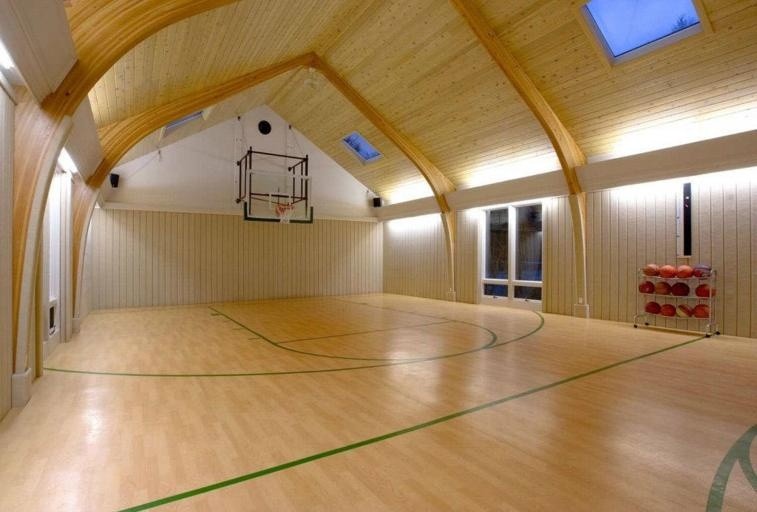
373,198,381,207
110,174,119,188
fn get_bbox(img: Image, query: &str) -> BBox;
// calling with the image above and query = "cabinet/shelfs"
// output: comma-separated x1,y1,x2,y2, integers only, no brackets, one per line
632,266,720,337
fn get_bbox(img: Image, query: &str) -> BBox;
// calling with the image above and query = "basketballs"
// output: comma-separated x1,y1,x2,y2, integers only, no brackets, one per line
676,304,693,318
693,265,711,280
660,304,676,317
696,284,716,300
693,304,710,318
644,263,659,276
639,280,655,294
676,265,693,280
659,265,676,278
645,302,660,313
654,282,671,294
671,282,690,296
